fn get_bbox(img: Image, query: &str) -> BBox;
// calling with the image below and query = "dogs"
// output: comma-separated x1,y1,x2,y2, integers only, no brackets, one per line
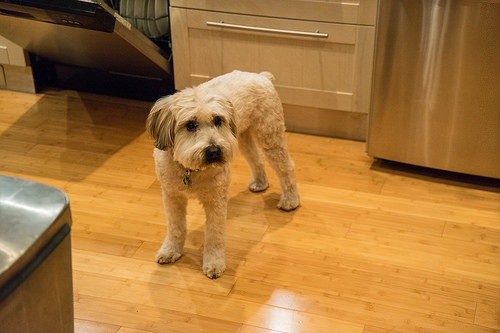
144,70,300,279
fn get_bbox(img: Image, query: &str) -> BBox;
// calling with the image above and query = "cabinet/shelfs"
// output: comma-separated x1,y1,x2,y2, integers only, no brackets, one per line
168,0,377,142
